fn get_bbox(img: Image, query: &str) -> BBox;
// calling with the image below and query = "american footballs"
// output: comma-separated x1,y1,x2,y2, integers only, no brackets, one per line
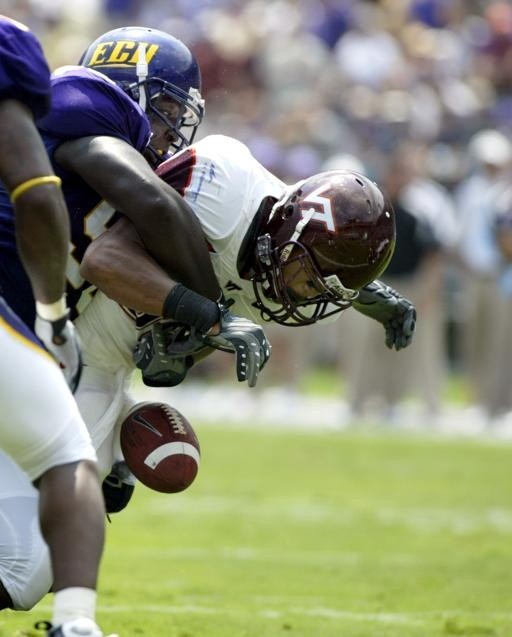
119,401,200,494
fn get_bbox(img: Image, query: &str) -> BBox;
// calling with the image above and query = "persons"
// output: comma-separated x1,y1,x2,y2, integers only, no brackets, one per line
1,1,512,429
0,16,416,636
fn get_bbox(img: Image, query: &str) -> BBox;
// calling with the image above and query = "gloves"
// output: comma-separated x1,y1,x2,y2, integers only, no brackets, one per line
30,306,84,397
163,285,273,380
352,280,428,360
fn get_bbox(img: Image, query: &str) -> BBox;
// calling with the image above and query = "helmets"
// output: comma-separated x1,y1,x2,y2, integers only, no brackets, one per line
65,20,206,165
0,17,53,95
265,156,412,296
